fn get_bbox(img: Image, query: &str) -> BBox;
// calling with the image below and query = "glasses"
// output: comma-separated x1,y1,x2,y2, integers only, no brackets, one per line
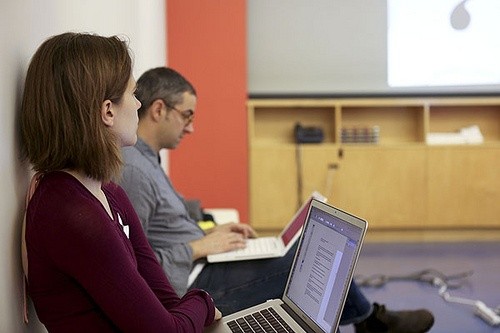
150,98,193,126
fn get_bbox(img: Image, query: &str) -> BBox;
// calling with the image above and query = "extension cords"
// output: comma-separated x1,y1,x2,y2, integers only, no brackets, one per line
472,300,500,326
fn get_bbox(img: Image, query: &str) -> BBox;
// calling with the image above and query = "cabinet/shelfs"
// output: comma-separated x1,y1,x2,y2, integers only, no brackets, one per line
246,96,500,243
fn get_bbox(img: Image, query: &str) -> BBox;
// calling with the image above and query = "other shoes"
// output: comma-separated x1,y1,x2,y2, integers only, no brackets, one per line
354,303,434,333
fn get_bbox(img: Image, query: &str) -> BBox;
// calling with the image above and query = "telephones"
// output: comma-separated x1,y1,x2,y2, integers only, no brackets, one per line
296,126,321,142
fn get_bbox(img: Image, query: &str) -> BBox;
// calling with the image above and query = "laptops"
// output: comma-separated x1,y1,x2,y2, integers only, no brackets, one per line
203,200,370,333
207,191,326,263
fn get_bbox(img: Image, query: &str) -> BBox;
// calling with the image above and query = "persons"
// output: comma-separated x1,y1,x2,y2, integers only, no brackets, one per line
109,66,435,333
18,32,222,333
